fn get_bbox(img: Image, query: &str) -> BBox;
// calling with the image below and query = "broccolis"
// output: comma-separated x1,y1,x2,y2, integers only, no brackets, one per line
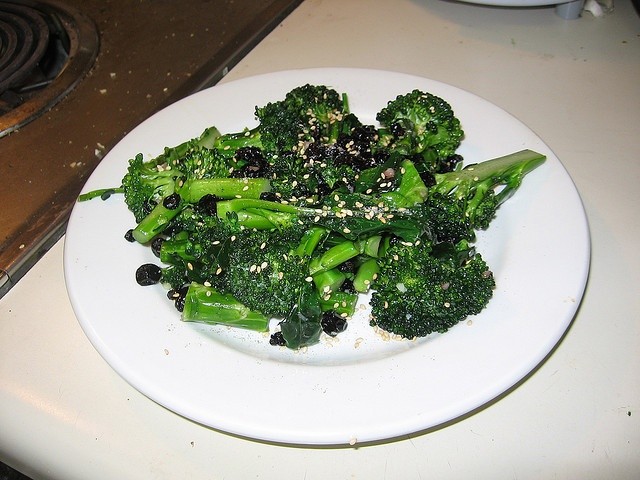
76,82,546,350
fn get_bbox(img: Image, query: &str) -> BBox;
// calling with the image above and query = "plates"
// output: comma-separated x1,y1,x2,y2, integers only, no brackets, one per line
63,68,590,445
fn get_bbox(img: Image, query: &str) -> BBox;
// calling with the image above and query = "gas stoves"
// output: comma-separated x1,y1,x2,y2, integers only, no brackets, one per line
0,0,304,298
